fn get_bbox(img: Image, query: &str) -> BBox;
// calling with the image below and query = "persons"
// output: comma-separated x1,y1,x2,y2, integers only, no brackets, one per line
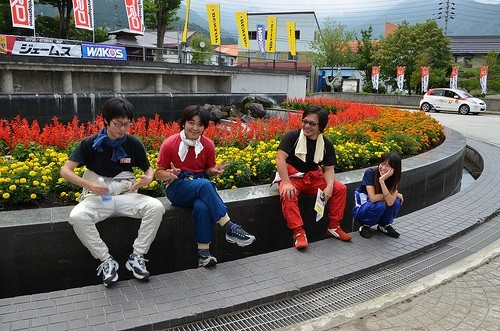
276,106,352,249
156,105,255,268
354,153,404,239
61,99,165,287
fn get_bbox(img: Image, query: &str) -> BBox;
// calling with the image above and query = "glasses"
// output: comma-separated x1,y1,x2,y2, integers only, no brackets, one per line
302,118,319,126
110,118,131,128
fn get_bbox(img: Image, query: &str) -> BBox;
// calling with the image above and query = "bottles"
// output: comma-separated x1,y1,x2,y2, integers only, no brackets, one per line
98,177,113,206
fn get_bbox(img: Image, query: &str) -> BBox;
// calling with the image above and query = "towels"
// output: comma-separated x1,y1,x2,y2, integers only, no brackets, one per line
294,129,325,164
177,129,204,162
92,127,127,152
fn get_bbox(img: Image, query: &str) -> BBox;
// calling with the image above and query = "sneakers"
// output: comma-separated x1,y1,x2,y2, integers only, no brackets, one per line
125,253,150,282
225,223,256,247
358,224,372,238
197,249,218,267
376,224,400,238
292,229,310,249
96,255,120,288
325,226,353,241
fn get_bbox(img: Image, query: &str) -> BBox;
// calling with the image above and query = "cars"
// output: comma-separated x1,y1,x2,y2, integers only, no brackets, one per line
419,88,487,115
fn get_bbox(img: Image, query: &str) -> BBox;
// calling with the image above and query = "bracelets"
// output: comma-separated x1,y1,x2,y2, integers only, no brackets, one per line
384,193,389,197
